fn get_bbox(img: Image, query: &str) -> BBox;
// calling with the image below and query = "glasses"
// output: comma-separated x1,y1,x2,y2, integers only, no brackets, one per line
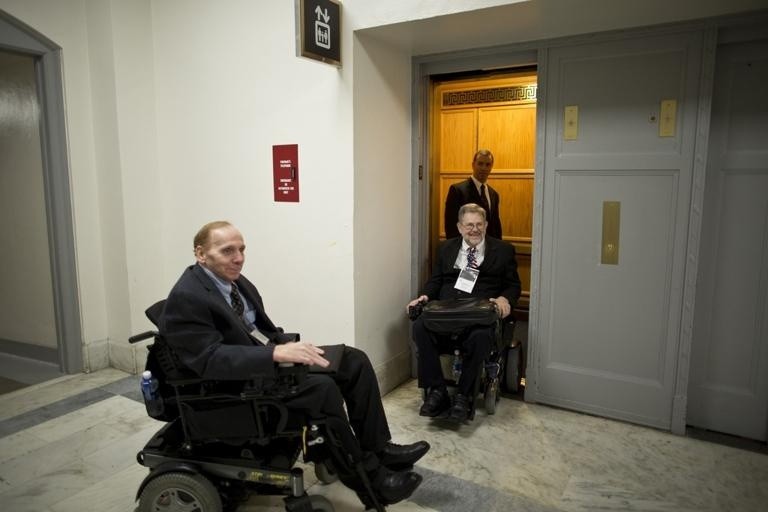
461,222,485,231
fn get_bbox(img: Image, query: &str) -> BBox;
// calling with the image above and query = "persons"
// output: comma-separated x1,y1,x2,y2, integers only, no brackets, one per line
444,149,504,242
159,220,430,509
405,203,522,425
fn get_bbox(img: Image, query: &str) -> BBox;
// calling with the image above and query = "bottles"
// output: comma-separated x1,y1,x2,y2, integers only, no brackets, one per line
141,371,165,417
452,350,462,378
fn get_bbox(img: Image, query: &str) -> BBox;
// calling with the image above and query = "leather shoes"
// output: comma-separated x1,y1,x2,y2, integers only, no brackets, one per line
373,440,430,471
418,385,452,416
355,468,423,509
450,393,471,423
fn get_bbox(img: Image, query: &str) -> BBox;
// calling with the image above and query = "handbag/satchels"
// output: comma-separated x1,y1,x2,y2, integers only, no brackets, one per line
422,298,499,332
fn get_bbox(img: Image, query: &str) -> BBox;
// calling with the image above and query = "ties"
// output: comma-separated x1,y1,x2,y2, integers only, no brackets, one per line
468,246,478,270
229,283,255,332
481,185,490,211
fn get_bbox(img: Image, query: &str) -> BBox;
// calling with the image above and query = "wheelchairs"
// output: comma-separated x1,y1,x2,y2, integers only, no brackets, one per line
124,297,387,512
404,284,525,422
